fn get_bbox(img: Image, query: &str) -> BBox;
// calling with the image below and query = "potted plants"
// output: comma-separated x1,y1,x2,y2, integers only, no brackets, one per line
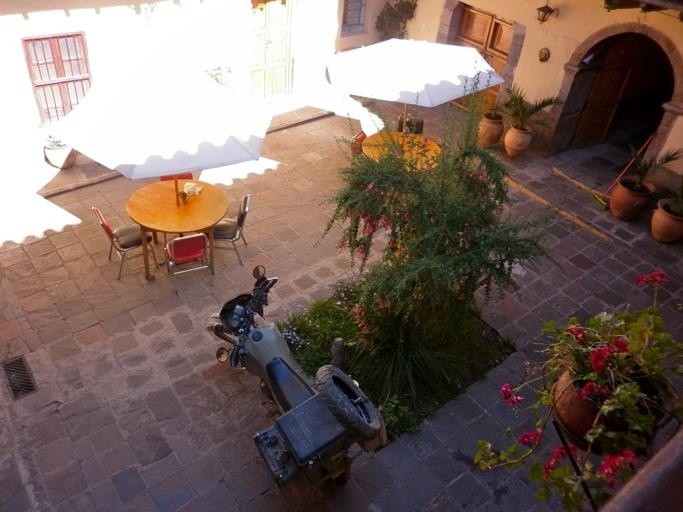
504,84,568,159
651,192,683,243
477,104,504,148
45,132,78,170
609,140,683,221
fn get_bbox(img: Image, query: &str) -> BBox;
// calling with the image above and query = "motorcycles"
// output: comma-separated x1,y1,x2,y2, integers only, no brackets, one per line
204,265,382,490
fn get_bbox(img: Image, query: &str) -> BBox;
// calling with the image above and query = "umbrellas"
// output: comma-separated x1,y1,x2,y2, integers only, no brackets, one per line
327,37,506,147
0,178,83,248
46,87,274,206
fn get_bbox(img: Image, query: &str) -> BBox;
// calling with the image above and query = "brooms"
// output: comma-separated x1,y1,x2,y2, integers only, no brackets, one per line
591,136,655,213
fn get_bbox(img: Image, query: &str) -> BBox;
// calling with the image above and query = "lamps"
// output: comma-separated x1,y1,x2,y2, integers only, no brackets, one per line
537,6,560,24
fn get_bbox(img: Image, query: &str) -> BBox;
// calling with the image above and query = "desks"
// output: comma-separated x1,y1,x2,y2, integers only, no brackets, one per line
362,133,442,171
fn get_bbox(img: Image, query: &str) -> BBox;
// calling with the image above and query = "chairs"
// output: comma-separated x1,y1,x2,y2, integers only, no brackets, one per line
351,131,367,155
92,173,251,295
398,116,423,135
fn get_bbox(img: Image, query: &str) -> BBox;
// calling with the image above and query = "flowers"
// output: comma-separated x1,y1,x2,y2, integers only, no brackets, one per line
475,271,683,512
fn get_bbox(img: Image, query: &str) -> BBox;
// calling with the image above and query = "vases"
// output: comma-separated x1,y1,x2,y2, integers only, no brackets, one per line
551,371,604,456
